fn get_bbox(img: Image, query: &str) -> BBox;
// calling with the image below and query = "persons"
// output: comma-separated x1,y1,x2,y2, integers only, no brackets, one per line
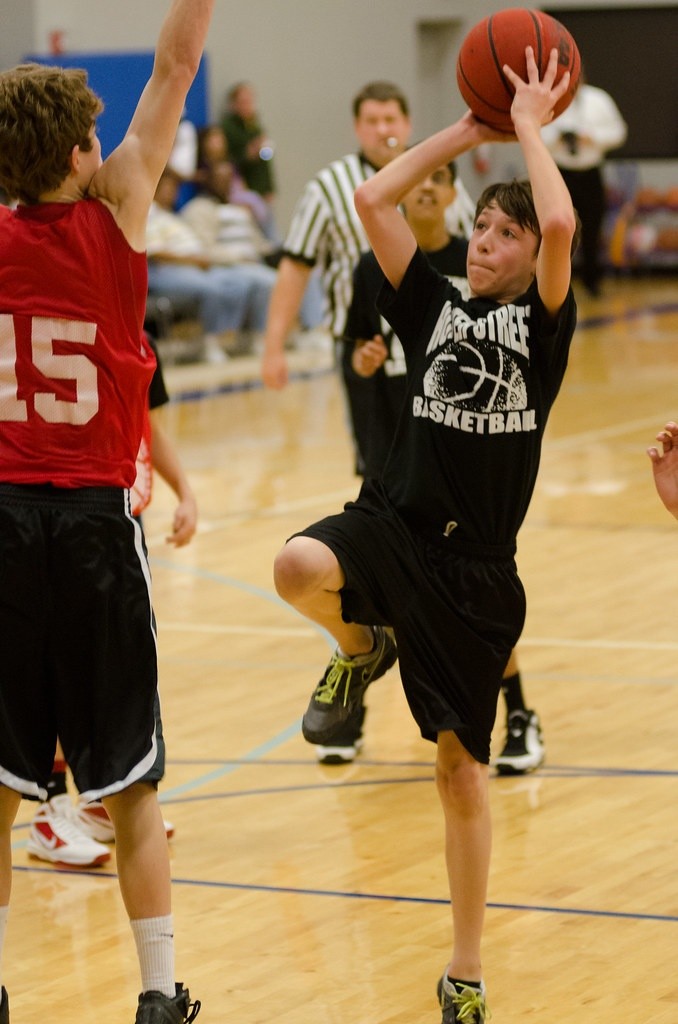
146,81,325,370
318,142,546,777
273,45,571,1024
0,0,211,1023
541,84,626,298
26,333,198,867
261,81,477,477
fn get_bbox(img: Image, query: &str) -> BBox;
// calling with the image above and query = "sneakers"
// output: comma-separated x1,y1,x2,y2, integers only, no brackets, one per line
135,980,201,1024
494,712,546,774
316,708,368,763
0,985,9,1024
77,799,175,842
437,961,492,1024
27,794,112,867
301,624,397,746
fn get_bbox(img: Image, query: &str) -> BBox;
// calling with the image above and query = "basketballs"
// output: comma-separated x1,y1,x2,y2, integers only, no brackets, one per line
456,8,582,131
